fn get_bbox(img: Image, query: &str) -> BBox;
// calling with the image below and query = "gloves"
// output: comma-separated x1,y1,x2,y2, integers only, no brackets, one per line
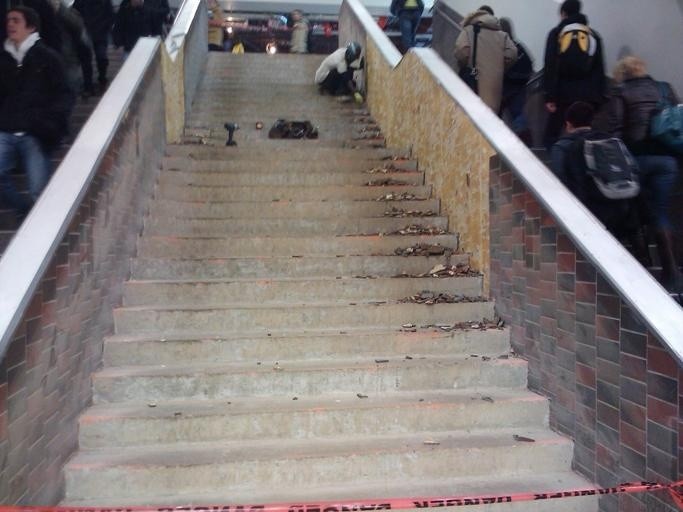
360,56,365,69
354,91,362,103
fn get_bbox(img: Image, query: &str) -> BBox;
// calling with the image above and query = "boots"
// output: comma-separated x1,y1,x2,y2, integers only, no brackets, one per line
627,224,651,268
656,222,683,295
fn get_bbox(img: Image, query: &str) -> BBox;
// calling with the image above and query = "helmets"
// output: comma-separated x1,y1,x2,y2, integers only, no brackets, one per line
347,42,361,59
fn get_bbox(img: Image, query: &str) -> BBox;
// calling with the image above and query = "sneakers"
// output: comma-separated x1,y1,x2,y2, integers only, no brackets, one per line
335,95,351,101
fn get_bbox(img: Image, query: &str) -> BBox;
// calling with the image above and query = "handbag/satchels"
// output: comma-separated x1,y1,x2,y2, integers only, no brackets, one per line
459,67,477,95
648,102,682,153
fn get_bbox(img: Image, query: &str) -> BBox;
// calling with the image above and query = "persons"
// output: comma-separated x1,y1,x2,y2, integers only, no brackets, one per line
493,18,533,148
594,54,682,297
547,100,630,243
388,0,424,49
287,9,311,55
1,1,170,211
540,1,606,151
207,0,229,51
452,5,519,117
314,41,365,104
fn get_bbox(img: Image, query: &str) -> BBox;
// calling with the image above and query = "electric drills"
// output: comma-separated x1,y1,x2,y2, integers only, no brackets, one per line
224,122,241,146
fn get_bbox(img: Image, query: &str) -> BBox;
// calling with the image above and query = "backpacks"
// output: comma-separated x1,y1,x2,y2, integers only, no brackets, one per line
561,131,642,202
